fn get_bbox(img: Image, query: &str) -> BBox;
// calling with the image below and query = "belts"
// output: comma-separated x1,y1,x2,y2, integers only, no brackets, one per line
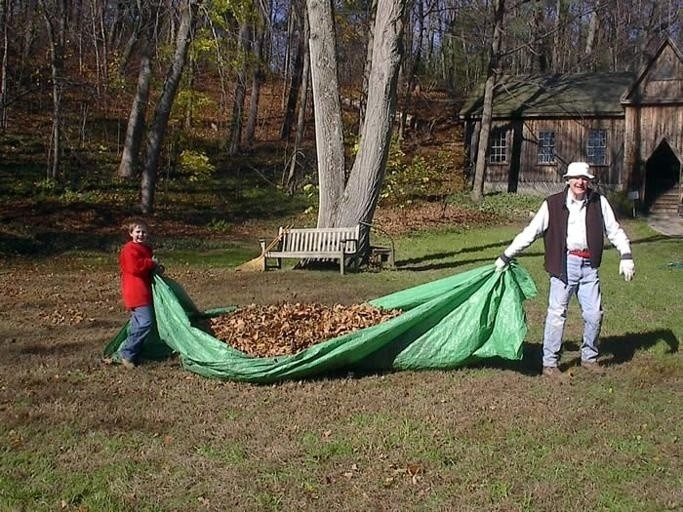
570,251,591,258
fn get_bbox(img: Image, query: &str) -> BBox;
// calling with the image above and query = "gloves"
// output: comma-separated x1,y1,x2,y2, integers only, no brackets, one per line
618,253,635,282
494,253,509,273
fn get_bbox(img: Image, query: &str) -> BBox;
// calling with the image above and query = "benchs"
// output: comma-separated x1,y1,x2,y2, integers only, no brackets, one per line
260,222,370,275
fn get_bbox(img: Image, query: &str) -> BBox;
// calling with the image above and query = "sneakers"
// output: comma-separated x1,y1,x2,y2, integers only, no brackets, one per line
580,361,605,376
121,356,134,371
542,365,562,380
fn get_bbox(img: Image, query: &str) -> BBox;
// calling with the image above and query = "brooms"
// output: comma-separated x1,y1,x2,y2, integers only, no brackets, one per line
233,205,315,273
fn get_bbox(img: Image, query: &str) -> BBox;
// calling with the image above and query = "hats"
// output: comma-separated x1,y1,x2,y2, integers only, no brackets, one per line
563,162,595,180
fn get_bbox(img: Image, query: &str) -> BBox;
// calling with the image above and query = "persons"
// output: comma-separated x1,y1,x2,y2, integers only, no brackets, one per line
116,219,165,370
494,160,635,380
